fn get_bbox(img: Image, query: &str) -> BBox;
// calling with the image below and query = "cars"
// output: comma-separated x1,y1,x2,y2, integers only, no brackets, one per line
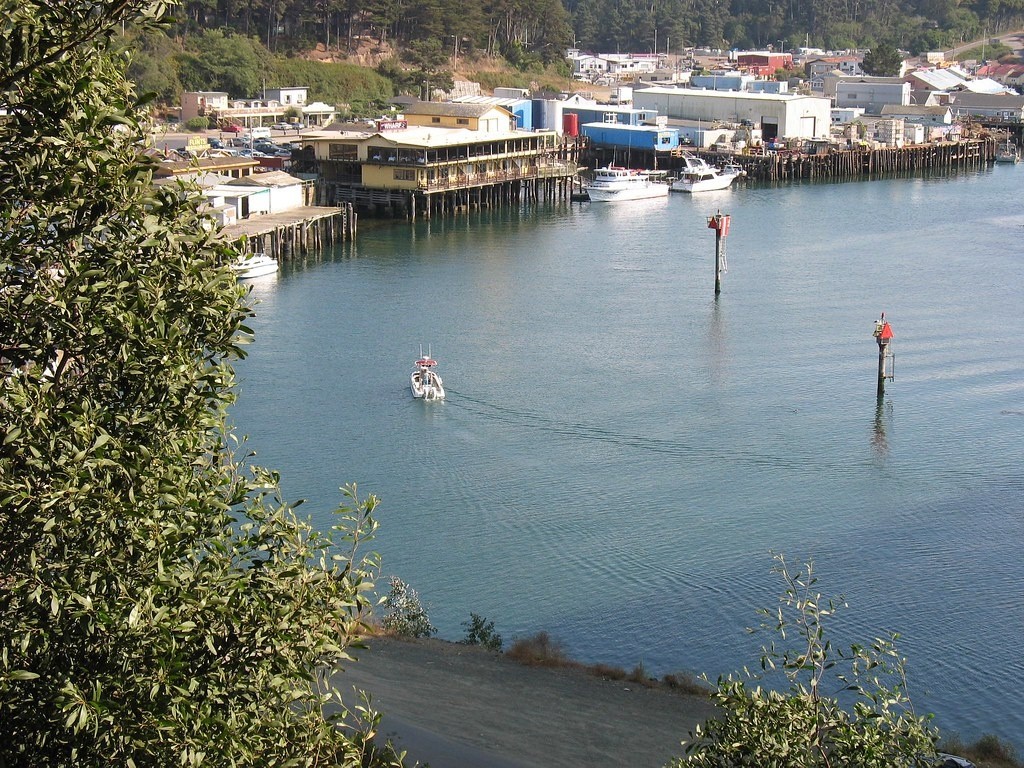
255,137,279,143
256,144,280,154
221,124,242,132
280,142,298,150
228,138,251,148
658,53,667,58
177,149,197,157
225,150,243,157
679,137,691,145
271,122,292,130
275,149,292,156
289,123,305,130
243,137,272,148
240,149,266,156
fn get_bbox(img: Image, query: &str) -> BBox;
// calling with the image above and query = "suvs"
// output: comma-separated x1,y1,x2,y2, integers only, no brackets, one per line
208,138,226,149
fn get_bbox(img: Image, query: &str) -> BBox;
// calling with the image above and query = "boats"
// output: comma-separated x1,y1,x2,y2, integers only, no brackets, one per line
581,161,670,202
409,343,445,401
722,165,747,181
670,156,738,194
232,253,279,278
996,127,1019,163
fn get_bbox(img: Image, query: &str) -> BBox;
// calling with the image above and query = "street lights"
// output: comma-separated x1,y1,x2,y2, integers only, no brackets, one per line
451,36,457,70
778,40,783,53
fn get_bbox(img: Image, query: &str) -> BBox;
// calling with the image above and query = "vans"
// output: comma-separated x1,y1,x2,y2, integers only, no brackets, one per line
244,128,271,138
